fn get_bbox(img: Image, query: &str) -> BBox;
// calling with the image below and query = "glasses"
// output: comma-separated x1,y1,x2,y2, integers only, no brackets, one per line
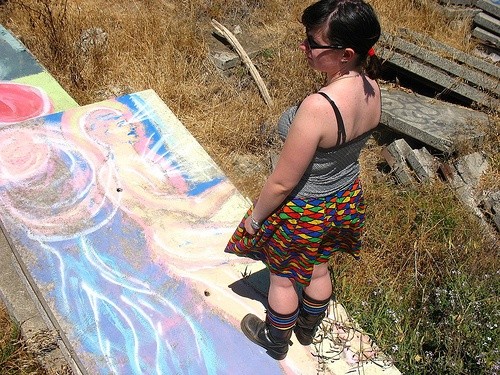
306,32,346,49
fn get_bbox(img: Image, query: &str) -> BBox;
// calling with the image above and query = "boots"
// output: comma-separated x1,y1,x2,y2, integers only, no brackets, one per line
295,318,323,346
241,313,293,360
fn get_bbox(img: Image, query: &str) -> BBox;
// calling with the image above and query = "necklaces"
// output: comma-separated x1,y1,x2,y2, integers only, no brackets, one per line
328,69,363,85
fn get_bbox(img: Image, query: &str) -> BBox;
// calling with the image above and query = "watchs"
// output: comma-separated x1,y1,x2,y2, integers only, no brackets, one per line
250,212,261,230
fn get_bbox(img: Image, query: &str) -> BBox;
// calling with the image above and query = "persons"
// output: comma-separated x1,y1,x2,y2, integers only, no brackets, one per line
225,0,383,360
